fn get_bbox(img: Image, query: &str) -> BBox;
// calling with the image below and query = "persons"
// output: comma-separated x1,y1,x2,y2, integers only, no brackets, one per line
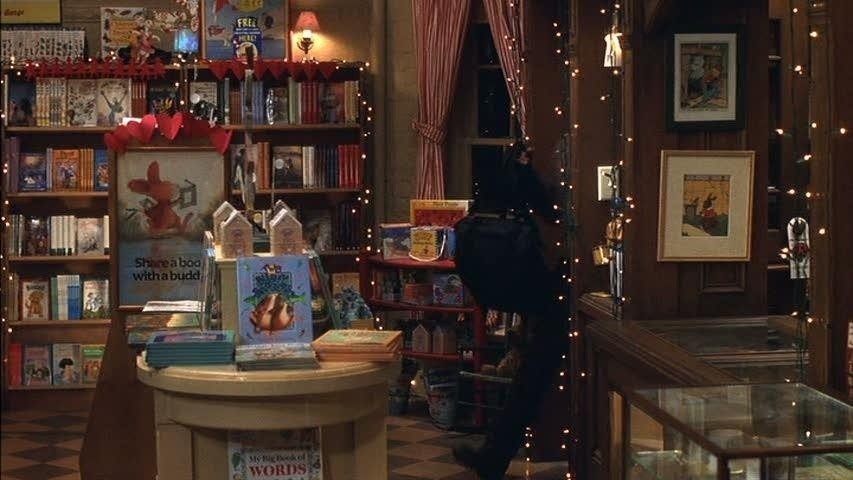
451,274,573,477
685,62,725,108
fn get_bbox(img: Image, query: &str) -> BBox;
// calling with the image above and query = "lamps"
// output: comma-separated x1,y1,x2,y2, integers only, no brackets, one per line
294,9,320,55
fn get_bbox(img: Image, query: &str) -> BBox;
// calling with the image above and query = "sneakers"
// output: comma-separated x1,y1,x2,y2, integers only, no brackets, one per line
452,440,504,478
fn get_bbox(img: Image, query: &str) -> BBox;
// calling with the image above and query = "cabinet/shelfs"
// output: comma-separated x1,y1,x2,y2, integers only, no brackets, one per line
133,343,396,479
370,257,485,426
2,61,362,428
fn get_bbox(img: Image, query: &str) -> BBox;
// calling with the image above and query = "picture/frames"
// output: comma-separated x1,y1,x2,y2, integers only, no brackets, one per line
654,148,757,266
670,32,744,128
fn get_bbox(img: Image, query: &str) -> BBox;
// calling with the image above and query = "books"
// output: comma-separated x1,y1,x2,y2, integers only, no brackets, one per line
123,247,404,370
8,76,180,128
5,272,110,321
5,214,110,258
8,342,105,385
3,136,108,194
333,272,361,311
188,76,359,126
292,198,360,252
230,142,363,191
1,25,86,67
